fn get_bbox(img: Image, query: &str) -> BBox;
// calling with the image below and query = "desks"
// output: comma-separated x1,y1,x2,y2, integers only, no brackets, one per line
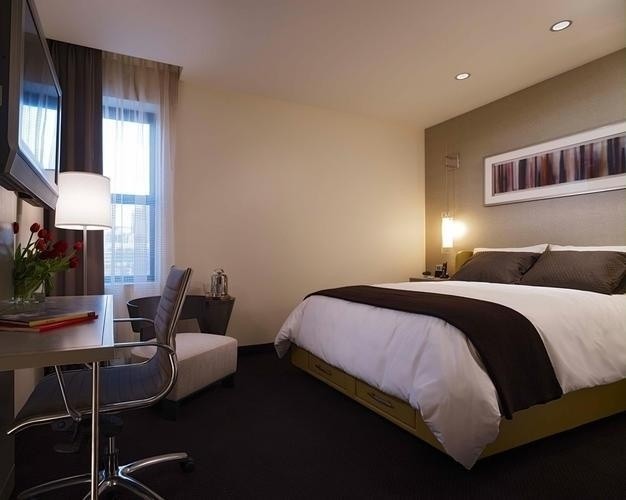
0,294,115,499
127,294,236,340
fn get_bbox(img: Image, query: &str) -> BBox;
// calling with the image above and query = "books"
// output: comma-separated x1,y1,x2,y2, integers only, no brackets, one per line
3,309,95,327
0,313,98,333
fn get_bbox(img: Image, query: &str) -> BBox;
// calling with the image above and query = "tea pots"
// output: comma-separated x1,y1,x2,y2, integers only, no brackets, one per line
200,266,230,301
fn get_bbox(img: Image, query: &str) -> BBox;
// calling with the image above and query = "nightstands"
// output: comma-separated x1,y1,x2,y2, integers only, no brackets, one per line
410,274,443,283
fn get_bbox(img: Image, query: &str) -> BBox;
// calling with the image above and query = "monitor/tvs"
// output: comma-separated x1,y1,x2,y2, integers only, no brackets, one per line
0,0,63,210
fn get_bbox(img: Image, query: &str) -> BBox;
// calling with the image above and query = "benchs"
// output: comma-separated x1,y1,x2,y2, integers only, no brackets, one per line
130,331,238,402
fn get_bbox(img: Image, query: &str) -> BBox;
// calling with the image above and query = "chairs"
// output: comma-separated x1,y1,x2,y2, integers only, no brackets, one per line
6,266,193,500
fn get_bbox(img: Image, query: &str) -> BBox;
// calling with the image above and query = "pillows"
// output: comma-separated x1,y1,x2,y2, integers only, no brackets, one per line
451,242,626,296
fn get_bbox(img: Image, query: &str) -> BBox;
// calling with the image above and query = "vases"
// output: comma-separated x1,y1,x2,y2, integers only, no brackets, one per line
10,277,45,312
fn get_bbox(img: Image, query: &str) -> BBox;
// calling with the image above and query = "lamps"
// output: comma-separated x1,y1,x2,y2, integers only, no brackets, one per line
54,171,113,293
440,145,462,251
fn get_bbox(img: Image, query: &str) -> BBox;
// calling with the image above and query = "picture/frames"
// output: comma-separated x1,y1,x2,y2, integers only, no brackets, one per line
481,120,626,208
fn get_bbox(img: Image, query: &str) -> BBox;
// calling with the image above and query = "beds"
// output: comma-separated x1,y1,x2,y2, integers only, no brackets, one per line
273,243,625,472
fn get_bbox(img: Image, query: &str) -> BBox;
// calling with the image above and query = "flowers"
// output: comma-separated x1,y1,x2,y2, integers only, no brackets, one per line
10,222,88,302
